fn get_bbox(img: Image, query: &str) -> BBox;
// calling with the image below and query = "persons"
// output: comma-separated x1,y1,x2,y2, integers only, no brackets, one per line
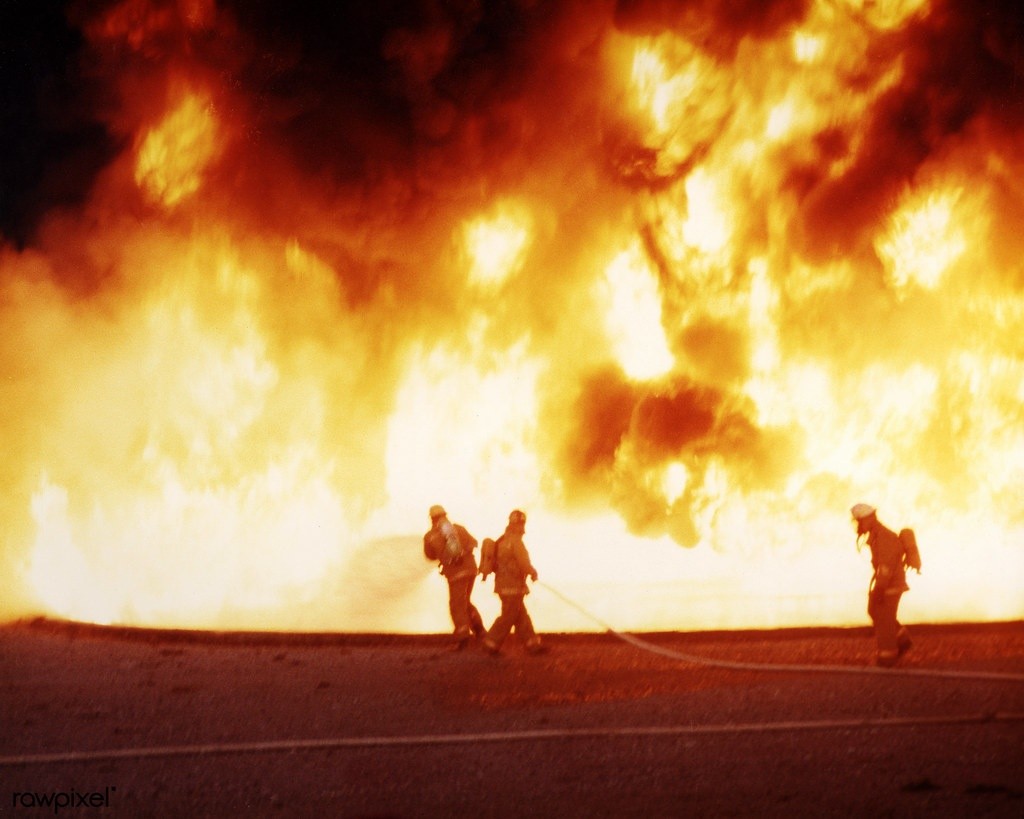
480,509,551,656
851,504,915,666
422,505,487,648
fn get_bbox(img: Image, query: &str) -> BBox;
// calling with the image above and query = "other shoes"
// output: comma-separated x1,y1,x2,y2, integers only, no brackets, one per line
877,657,895,668
525,645,552,655
898,638,913,656
481,643,504,656
447,637,467,653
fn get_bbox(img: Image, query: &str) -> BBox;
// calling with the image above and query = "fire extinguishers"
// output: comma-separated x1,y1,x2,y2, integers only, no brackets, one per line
437,515,467,563
478,538,496,578
899,530,922,571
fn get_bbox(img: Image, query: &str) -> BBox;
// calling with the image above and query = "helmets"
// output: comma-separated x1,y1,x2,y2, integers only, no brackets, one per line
508,510,526,524
430,505,446,519
852,504,877,519
